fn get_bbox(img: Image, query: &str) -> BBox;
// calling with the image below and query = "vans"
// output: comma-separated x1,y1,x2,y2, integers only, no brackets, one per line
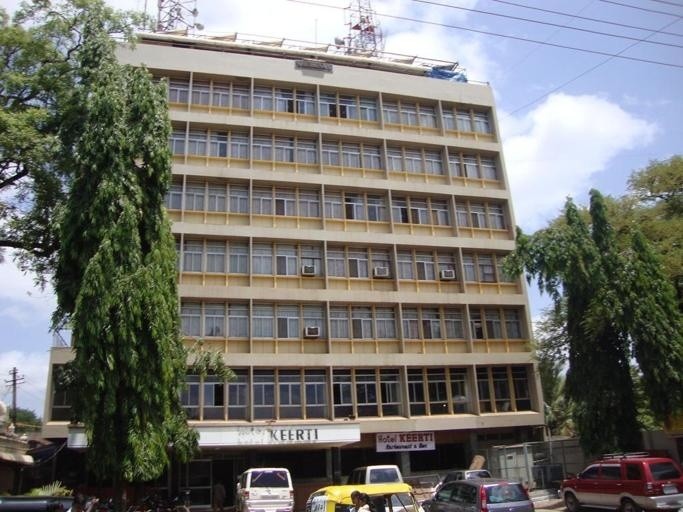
344,465,406,483
304,484,423,512
234,467,294,511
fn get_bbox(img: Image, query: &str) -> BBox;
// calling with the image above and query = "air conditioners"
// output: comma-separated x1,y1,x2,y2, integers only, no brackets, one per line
301,264,316,276
373,266,389,278
440,270,455,280
304,326,320,338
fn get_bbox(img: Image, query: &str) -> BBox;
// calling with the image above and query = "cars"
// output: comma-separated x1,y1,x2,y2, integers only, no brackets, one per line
435,469,492,492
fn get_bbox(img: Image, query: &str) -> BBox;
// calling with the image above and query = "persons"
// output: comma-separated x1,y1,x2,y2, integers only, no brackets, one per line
355,493,371,512
347,491,361,511
211,479,226,512
66,492,85,512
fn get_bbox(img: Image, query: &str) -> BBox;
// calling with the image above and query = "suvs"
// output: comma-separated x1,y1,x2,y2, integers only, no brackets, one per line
420,478,537,512
555,450,682,512
